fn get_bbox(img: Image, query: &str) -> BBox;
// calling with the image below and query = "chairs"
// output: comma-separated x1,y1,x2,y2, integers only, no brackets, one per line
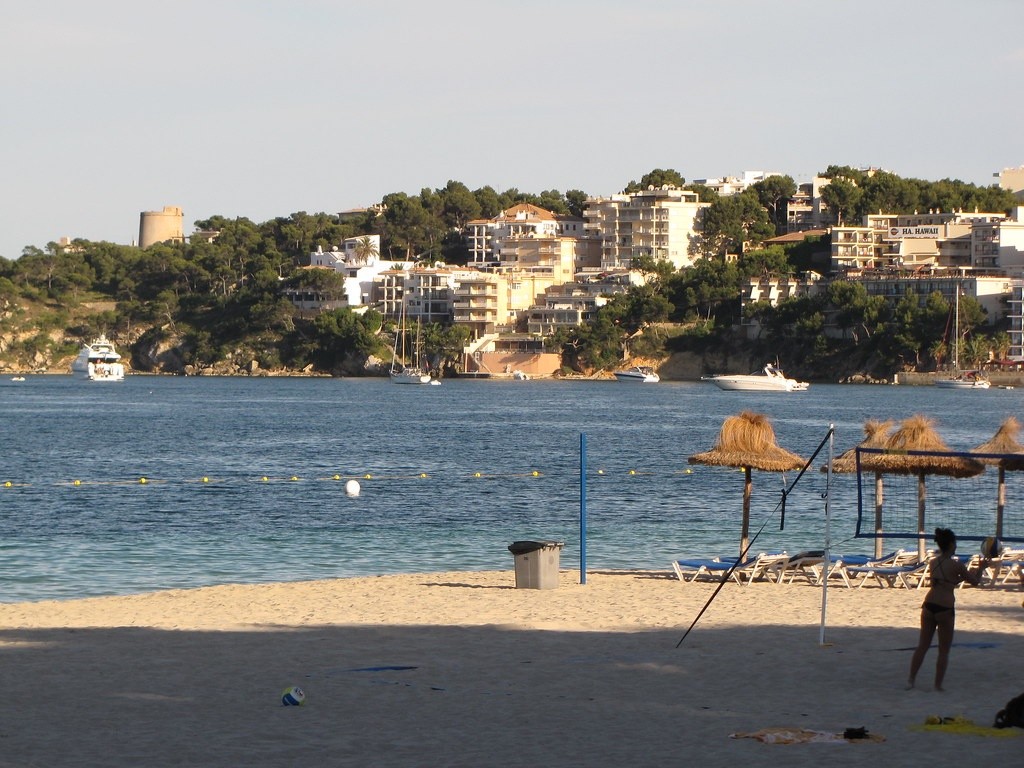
672,549,1024,592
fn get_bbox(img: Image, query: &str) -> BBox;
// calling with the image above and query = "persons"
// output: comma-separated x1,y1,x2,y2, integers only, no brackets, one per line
905,527,995,691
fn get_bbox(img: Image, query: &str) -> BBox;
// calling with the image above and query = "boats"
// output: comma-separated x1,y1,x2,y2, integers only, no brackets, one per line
701,355,810,392
613,366,661,383
10,376,25,381
71,334,126,382
998,384,1014,389
513,370,530,380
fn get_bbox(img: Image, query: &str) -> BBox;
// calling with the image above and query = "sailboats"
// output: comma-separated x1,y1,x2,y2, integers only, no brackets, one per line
931,281,991,389
387,277,431,385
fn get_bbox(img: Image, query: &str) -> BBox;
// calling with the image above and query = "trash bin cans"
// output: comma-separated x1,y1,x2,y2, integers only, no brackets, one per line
512,539,564,589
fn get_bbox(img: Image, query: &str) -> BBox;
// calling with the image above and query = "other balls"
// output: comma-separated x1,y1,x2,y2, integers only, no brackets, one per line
980,537,1003,558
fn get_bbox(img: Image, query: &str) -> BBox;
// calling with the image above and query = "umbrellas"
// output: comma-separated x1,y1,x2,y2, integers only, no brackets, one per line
689,410,1024,566
